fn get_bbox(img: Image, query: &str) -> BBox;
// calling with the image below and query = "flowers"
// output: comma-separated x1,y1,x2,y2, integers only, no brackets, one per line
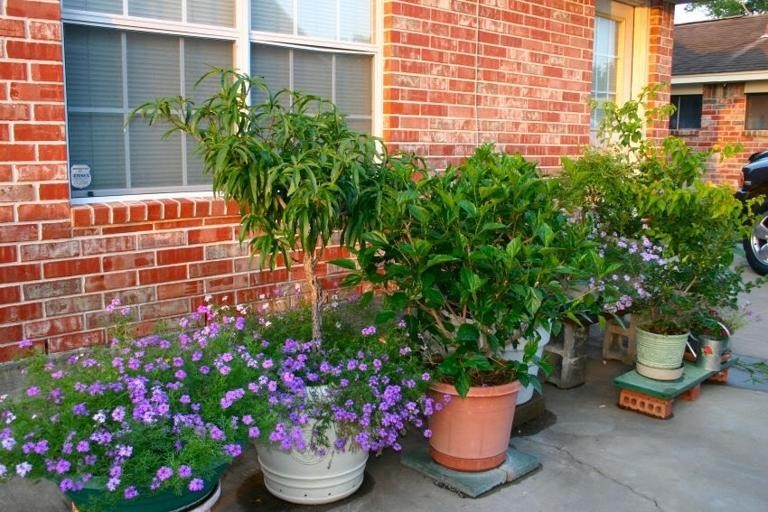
178,283,452,456
0,298,245,512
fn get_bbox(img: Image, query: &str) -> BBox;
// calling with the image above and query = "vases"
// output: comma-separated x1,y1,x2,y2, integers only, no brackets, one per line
253,385,371,505
45,453,229,512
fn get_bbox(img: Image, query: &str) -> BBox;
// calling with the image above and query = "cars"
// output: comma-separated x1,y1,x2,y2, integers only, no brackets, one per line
734,151,768,275
